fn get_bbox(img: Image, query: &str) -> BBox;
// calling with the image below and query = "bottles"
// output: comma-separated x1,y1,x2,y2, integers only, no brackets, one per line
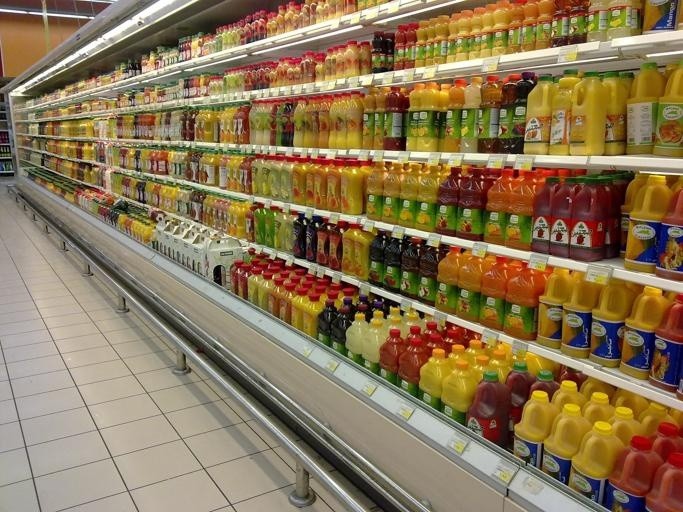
335,92,351,149
367,229,391,287
0,131,14,172
491,0,510,57
459,76,484,153
361,160,374,213
258,272,276,312
346,90,364,150
318,94,333,148
397,162,424,229
468,6,486,61
362,88,380,150
294,96,308,147
444,79,467,152
478,335,560,385
521,0,644,52
293,212,310,259
247,268,264,305
414,164,441,232
483,168,519,246
399,237,426,300
305,215,323,263
341,222,361,276
406,83,426,151
326,158,345,212
373,86,391,150
297,0,394,86
405,23,417,69
383,86,406,151
417,245,446,307
291,157,311,204
341,160,364,215
230,259,244,295
414,20,428,68
291,287,310,331
314,158,330,210
16,0,297,255
356,230,375,282
510,71,535,154
479,255,509,331
480,3,498,58
502,260,539,340
384,231,408,292
367,160,389,221
432,14,451,65
497,74,522,154
416,82,440,152
279,283,297,326
315,218,335,266
379,303,478,426
237,263,253,299
509,0,521,54
304,95,320,148
267,277,286,318
434,245,462,314
456,9,473,62
438,84,452,152
455,256,485,322
381,162,405,224
456,168,492,241
328,220,348,271
302,278,387,376
434,166,464,237
477,74,503,153
328,93,346,149
394,24,406,70
445,12,461,64
305,158,322,207
504,171,539,252
425,18,438,66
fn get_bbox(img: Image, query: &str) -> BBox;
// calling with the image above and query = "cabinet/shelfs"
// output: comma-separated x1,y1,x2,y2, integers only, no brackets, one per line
0,0,681,512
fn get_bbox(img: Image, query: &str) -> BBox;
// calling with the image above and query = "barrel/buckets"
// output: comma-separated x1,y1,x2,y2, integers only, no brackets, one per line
522,73,554,153
607,405,641,447
513,390,558,469
654,187,683,281
606,433,663,512
581,392,615,428
567,420,625,508
580,375,614,404
611,387,649,421
551,379,587,413
504,361,530,447
466,369,508,450
620,173,649,257
653,60,683,156
645,453,683,512
647,293,683,391
536,266,571,348
619,286,670,380
568,70,607,155
625,62,667,155
623,173,673,273
540,402,593,486
638,401,679,435
560,270,599,359
528,369,561,402
557,364,585,390
670,174,683,191
648,421,680,463
600,71,629,156
588,278,636,368
